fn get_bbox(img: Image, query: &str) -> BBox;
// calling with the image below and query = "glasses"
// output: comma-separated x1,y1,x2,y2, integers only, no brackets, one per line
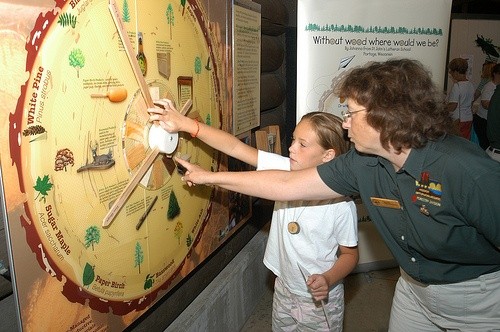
340,108,368,121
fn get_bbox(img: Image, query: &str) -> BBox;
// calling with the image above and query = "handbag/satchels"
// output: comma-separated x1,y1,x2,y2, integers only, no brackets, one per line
454,119,461,135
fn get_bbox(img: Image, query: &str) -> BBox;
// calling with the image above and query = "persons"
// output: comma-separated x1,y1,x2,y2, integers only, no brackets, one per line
447,58,474,139
148,98,359,332
175,57,500,332
486,83,500,163
474,62,497,99
472,63,500,151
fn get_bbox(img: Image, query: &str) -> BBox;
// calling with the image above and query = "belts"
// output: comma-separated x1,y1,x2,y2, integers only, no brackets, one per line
489,147,500,154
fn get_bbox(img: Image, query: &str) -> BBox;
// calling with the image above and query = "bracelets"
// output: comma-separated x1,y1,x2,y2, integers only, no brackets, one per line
288,200,311,233
190,119,199,138
